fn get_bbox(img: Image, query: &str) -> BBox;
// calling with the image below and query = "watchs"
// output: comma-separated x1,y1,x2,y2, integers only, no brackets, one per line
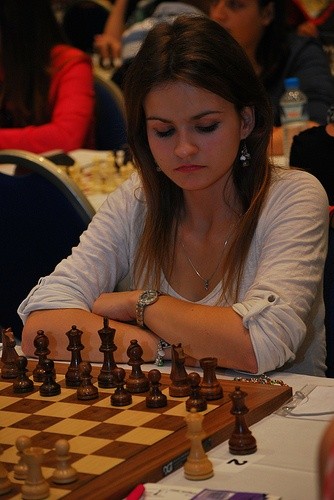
135,289,170,327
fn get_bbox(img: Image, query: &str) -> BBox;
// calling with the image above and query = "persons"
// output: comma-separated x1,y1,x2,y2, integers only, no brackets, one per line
0,0,334,343
18,13,330,377
318,416,334,500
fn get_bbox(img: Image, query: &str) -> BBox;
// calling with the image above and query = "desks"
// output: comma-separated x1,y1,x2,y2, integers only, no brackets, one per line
0,342,334,500
44,150,137,213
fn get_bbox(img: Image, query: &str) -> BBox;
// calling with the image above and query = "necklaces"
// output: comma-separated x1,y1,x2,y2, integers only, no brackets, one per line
174,228,233,295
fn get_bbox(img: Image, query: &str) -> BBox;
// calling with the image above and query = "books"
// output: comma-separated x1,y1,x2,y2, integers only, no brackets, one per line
126,481,282,500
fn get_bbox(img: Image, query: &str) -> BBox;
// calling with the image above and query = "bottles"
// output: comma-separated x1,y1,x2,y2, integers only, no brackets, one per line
279,78,308,164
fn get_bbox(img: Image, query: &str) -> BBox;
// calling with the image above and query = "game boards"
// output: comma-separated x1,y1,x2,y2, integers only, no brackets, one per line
0,357,295,500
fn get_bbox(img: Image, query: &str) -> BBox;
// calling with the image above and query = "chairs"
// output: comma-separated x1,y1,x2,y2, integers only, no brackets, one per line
0,149,97,343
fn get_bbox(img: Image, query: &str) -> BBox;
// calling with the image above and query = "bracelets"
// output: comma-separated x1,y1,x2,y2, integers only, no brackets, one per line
154,338,172,366
